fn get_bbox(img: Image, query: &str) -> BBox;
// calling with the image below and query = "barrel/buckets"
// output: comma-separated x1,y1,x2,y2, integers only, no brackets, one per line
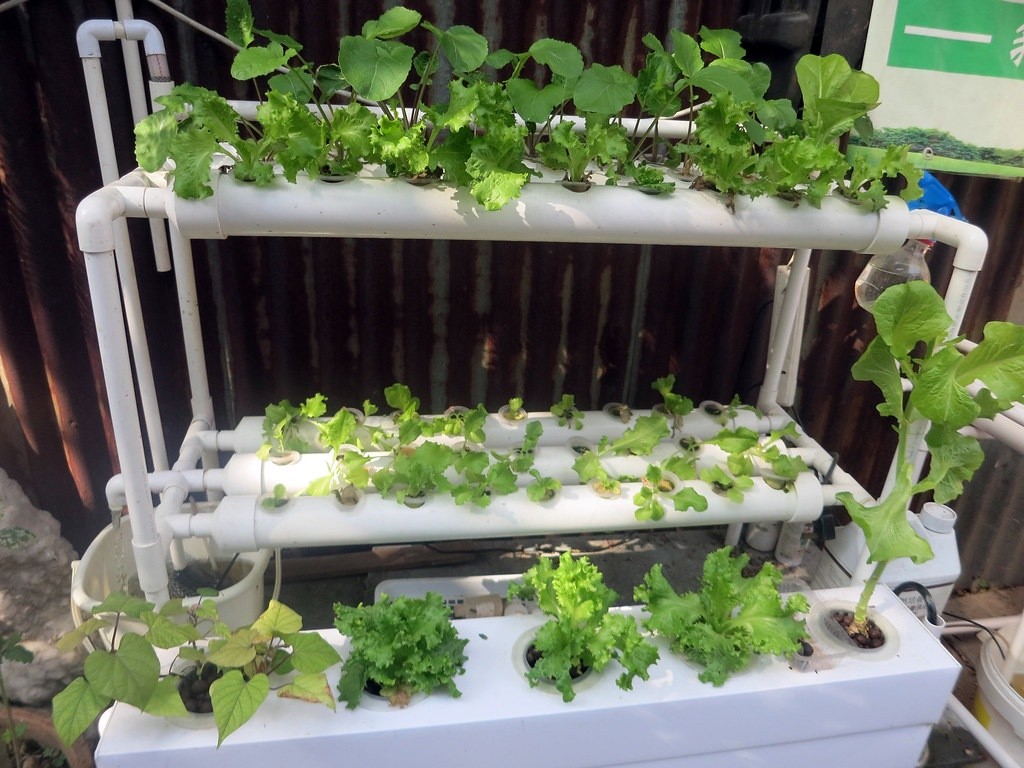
976,622,1024,768
71,500,281,653
809,501,962,618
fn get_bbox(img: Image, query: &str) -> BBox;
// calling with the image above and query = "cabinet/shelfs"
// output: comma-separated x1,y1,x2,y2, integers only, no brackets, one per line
74,98,988,591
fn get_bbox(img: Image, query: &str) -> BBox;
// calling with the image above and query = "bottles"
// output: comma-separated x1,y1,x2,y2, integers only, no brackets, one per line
775,521,813,566
